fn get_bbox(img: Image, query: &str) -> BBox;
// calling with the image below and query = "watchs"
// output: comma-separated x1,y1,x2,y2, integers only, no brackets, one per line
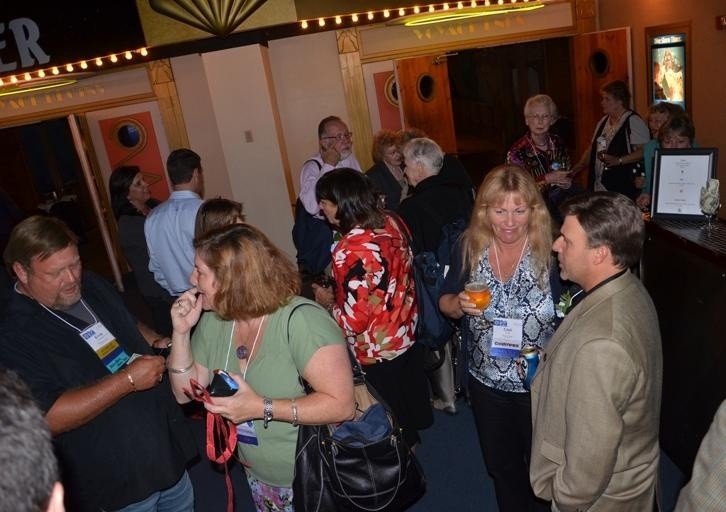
264,398,274,428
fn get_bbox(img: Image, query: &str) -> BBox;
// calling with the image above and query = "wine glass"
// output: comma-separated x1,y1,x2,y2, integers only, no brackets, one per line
699,187,721,232
464,277,493,331
598,151,610,171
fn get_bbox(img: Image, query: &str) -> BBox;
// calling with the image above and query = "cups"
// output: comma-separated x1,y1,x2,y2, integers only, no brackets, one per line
316,274,332,289
376,194,389,209
556,162,571,172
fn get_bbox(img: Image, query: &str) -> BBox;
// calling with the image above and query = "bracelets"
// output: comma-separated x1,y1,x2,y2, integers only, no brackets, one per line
291,398,298,427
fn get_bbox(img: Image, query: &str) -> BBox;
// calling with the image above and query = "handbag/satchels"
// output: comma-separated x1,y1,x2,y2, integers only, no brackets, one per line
288,192,334,279
289,373,430,512
618,140,645,177
410,245,455,354
